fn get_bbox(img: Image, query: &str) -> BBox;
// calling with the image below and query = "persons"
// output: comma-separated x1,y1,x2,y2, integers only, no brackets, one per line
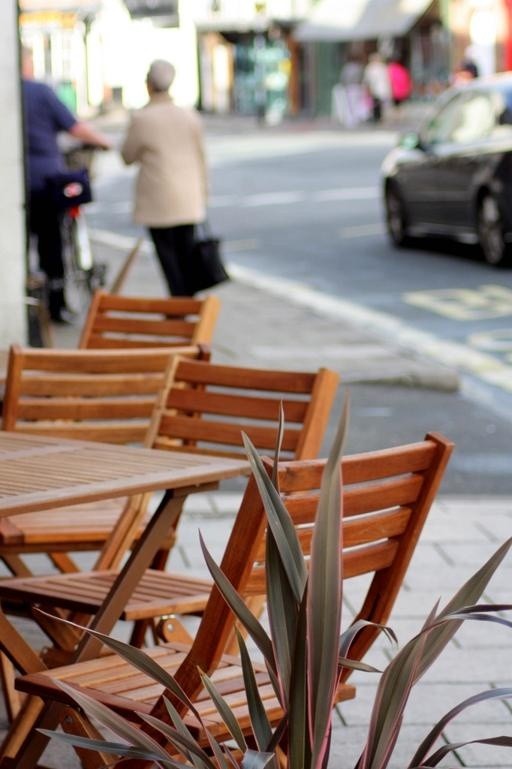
327,21,482,130
121,53,211,319
20,41,108,326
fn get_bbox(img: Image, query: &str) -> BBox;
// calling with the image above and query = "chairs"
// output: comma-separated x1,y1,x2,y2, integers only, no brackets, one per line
3,356,341,669
76,291,219,351
14,431,453,766
1,345,212,577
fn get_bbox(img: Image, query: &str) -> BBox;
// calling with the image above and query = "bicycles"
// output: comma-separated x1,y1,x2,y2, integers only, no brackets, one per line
60,142,109,296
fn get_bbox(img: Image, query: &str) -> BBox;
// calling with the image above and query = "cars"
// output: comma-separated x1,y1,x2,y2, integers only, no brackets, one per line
378,70,512,269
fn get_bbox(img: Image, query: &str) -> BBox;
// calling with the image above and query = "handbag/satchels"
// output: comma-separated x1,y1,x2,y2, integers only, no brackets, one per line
186,223,230,297
38,165,93,214
